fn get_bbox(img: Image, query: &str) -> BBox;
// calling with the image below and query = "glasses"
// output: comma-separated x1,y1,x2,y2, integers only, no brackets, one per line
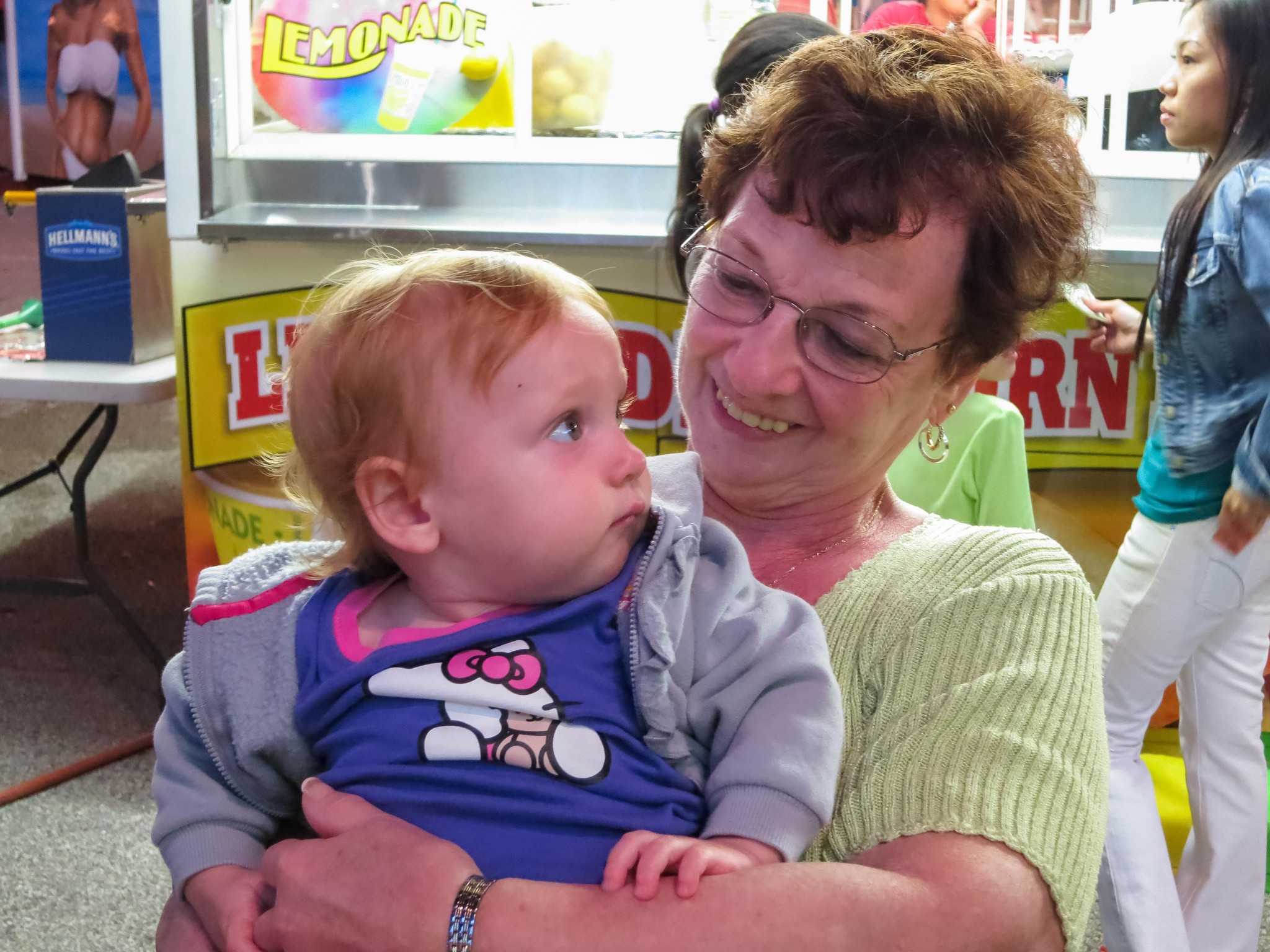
681,215,971,384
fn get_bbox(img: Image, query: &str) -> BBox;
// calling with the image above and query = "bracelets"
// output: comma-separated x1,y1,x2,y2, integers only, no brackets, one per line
446,874,497,952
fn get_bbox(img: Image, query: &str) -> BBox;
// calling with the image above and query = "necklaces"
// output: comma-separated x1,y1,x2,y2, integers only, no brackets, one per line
767,486,891,588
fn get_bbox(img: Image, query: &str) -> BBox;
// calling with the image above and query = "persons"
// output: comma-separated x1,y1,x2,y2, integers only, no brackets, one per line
45,0,152,180
859,0,997,53
148,10,1111,952
1081,0,1270,952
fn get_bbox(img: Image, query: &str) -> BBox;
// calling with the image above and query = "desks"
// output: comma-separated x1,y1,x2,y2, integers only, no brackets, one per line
0,309,180,667
